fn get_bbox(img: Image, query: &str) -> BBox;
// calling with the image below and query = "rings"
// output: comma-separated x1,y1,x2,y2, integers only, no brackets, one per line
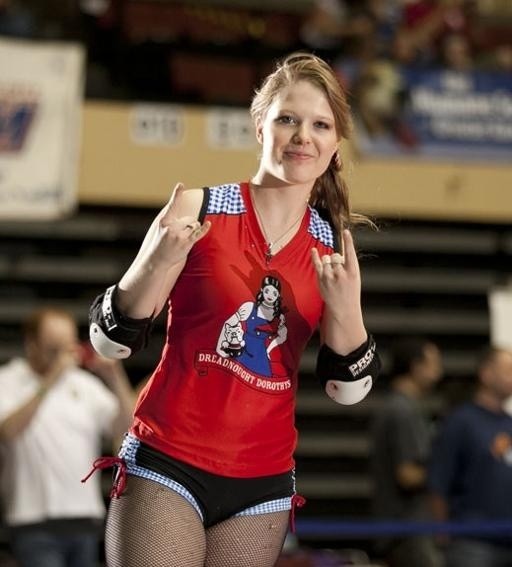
321,255,331,264
186,223,197,231
331,255,342,264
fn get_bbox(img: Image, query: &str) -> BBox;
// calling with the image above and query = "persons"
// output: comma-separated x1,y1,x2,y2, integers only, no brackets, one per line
0,304,137,567
371,336,452,567
421,345,511,567
295,1,511,149
86,54,383,567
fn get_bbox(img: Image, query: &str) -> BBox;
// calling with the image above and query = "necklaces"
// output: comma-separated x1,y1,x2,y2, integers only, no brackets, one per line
250,179,307,265
